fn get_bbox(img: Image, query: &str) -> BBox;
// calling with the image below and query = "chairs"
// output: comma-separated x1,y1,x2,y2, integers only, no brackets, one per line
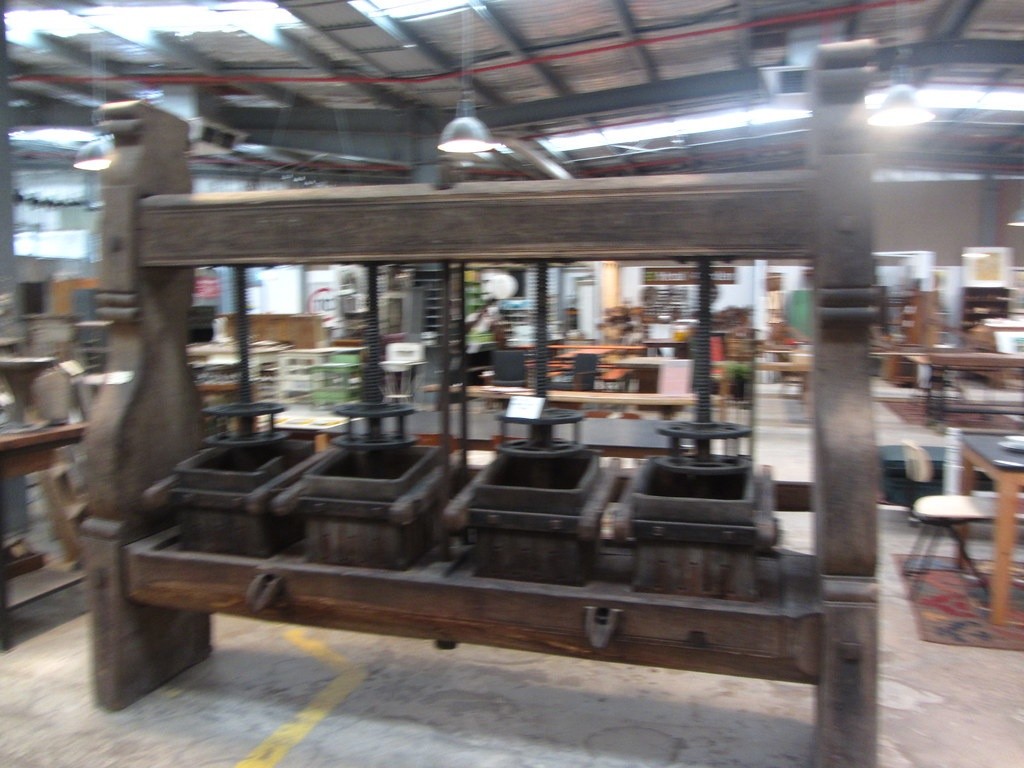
898,440,1000,600
491,349,530,407
551,353,602,390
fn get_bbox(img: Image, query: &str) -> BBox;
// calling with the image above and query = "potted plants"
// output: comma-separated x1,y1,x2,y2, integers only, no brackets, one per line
726,360,749,402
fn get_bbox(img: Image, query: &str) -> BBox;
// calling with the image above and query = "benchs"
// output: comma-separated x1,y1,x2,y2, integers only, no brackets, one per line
597,367,634,391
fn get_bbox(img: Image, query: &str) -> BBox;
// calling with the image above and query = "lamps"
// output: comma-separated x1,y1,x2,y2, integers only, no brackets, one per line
864,0,937,126
73,32,118,172
437,0,495,155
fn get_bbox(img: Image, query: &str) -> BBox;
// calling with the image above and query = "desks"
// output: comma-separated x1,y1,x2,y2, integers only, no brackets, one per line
277,347,341,394
421,382,728,452
547,344,648,365
957,433,1024,627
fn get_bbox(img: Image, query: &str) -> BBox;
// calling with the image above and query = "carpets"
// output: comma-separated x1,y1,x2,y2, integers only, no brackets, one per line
892,552,1024,651
881,397,1024,430
